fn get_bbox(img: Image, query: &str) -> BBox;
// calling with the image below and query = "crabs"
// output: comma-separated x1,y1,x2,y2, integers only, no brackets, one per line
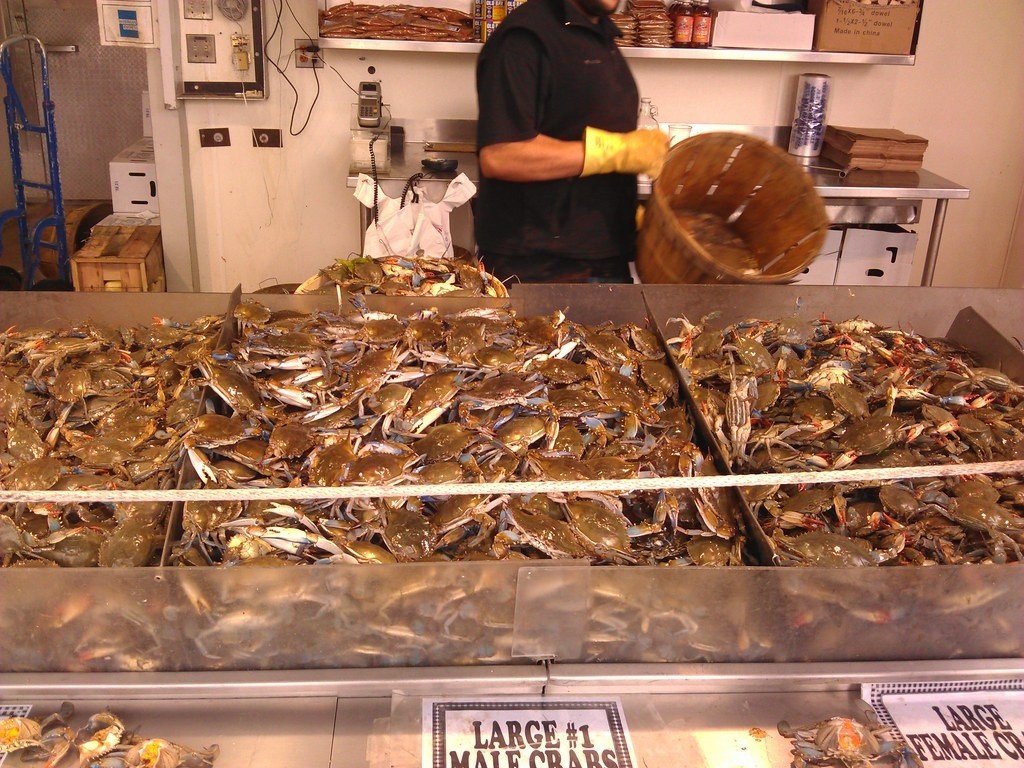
776,697,923,768
664,308,1024,662
0,293,750,671
0,701,219,768
301,257,505,298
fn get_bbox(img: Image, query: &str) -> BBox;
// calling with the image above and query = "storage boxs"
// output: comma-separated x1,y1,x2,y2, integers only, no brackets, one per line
67,89,161,292
711,10,816,50
473,0,528,43
815,2,920,53
787,226,920,284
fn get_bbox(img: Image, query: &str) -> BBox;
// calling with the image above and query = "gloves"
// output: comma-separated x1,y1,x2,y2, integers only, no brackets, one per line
635,207,649,231
579,127,671,182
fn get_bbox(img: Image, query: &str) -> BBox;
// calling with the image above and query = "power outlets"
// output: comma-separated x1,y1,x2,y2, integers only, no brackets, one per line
293,37,324,70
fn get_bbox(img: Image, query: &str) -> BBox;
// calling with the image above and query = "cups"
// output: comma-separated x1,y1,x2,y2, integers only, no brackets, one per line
667,124,693,149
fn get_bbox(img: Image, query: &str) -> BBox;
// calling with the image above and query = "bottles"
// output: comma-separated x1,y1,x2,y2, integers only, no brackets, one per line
635,97,660,131
667,0,712,49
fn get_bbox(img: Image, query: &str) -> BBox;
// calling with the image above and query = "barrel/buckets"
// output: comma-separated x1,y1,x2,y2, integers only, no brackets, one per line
634,130,829,285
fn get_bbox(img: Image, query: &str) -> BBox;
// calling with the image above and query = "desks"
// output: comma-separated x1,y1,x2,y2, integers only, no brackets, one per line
345,133,971,289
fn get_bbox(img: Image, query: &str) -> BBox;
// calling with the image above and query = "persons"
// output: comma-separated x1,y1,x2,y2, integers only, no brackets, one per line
475,0,671,283
